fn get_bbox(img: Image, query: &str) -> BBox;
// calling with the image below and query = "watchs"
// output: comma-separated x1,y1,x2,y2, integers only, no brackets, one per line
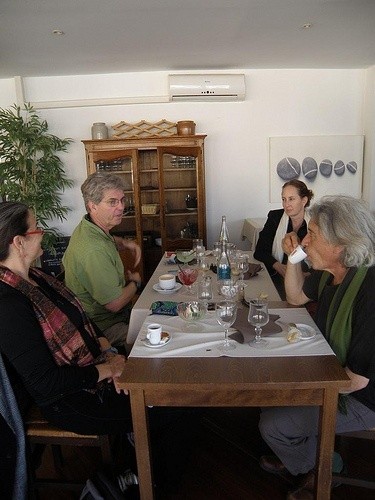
128,280,141,289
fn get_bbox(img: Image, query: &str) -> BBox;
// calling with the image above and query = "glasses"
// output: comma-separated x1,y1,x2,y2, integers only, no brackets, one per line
95,195,129,207
9,226,44,244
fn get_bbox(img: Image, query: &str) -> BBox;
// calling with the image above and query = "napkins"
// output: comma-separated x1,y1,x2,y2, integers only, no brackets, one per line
219,304,283,345
240,261,262,280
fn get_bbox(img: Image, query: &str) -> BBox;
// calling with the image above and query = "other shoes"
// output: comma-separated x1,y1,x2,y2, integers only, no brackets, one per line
288,466,349,500
86,466,139,500
257,454,291,476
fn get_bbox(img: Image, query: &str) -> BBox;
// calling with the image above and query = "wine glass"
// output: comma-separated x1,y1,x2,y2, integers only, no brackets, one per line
214,300,237,351
197,278,214,319
247,297,270,350
174,248,208,331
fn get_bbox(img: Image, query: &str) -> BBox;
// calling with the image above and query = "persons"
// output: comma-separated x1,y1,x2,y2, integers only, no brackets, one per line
62,170,142,357
253,179,313,298
256,195,375,484
0,201,173,500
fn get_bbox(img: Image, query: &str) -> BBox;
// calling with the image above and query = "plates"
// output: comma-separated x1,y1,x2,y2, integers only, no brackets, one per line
142,331,172,347
287,324,316,340
186,208,198,210
153,282,182,294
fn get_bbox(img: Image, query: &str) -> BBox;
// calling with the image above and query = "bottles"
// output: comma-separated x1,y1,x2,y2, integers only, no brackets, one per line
99,158,122,171
171,156,196,168
92,123,108,140
219,216,231,242
176,121,196,136
216,240,231,295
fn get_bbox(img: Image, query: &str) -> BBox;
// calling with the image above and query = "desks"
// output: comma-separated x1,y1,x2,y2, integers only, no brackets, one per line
240,217,268,252
116,252,351,500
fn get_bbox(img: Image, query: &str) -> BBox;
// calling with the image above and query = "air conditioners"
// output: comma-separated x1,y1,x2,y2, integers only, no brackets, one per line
167,74,246,102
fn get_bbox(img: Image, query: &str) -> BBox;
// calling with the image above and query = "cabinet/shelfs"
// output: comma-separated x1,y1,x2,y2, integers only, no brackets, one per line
81,135,208,281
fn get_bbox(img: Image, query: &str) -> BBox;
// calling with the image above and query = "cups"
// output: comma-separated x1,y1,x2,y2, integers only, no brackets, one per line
159,274,176,290
193,238,211,281
215,242,249,301
288,245,308,265
146,323,162,345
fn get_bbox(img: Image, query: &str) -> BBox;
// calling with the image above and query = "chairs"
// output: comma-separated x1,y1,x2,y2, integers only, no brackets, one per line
0,352,111,500
332,427,375,489
